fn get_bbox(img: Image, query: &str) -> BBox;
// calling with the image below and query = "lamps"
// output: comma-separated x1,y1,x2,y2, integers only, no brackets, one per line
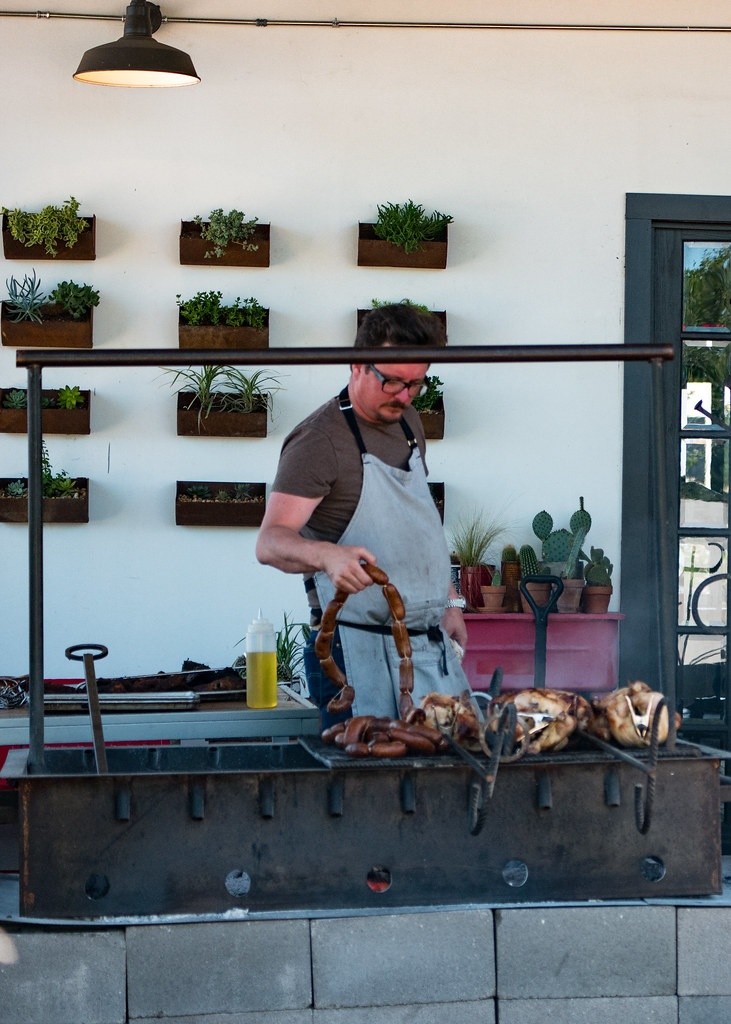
72,0,203,89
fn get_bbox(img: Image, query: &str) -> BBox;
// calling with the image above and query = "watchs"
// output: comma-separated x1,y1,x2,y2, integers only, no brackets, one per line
445,596,467,610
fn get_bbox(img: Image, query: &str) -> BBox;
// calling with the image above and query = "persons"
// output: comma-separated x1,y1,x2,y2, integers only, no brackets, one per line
255,304,486,723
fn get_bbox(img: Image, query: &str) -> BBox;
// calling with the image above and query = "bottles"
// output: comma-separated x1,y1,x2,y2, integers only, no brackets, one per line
244,608,278,708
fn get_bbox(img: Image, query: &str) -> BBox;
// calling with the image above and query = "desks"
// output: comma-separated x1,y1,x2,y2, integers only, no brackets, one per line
0,679,324,881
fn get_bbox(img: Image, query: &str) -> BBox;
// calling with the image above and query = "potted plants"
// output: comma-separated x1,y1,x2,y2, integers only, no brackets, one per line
444,495,616,615
0,205,458,530
229,616,316,703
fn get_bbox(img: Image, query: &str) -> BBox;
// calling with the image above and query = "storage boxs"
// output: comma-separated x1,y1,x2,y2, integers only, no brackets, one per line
457,609,625,693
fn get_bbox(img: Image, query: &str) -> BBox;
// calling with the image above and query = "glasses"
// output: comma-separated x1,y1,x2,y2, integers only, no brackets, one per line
370,365,432,398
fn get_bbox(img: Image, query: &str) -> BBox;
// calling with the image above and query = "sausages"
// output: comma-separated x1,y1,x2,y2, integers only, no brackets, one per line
314,564,452,758
